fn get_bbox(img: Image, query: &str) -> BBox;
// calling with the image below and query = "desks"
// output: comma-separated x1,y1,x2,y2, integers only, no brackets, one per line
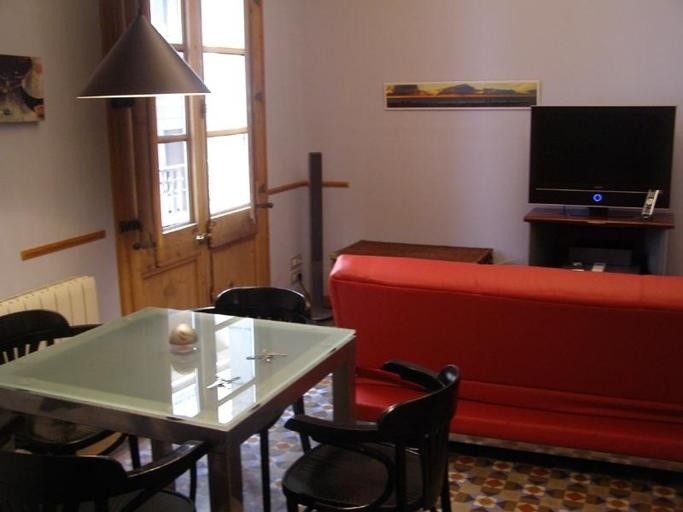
0,305,356,512
330,240,492,269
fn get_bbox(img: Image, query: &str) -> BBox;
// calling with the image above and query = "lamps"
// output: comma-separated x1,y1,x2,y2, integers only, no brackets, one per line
77,0,208,97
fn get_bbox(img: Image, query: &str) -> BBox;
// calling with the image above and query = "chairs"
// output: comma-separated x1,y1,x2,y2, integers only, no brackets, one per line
174,287,310,512
1,309,140,471
0,438,208,512
281,362,460,512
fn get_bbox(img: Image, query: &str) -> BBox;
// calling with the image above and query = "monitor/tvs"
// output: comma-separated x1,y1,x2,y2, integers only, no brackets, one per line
529,106,675,217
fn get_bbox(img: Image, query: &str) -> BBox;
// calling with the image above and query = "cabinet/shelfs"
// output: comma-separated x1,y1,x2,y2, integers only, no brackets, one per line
522,206,675,277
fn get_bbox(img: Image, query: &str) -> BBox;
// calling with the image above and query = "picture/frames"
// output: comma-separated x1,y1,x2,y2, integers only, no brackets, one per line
381,79,541,111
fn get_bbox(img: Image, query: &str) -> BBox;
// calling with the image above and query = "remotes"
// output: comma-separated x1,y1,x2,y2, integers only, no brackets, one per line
641,189,660,222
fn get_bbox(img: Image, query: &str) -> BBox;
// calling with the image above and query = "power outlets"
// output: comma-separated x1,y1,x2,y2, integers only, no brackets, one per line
290,268,302,287
289,254,301,271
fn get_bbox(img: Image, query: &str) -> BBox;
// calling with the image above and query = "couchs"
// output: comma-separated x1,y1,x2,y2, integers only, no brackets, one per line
326,251,682,473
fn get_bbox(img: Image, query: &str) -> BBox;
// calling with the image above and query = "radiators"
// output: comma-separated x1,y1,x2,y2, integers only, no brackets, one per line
0,275,99,343
